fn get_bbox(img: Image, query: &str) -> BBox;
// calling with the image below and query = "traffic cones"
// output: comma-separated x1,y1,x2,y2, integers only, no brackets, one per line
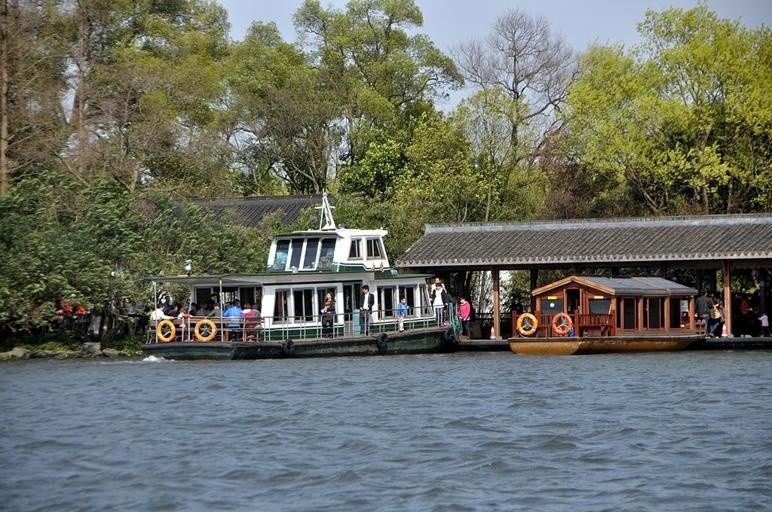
721,323,727,336
490,326,496,339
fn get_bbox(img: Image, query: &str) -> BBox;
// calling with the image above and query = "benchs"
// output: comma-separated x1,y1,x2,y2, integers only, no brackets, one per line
149,325,263,343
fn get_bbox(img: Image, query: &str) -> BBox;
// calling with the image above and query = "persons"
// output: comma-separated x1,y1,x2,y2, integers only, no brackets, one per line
695,288,771,339
396,295,410,332
430,276,448,297
149,293,261,342
458,296,472,339
509,296,522,316
432,283,445,326
359,285,374,336
322,292,336,338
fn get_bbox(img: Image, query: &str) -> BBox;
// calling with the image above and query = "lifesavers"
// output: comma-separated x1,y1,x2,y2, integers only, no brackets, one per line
156,319,176,343
517,313,538,335
194,319,217,342
551,313,573,334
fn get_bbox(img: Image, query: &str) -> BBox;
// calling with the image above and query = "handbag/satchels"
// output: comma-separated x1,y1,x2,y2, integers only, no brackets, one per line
710,305,721,319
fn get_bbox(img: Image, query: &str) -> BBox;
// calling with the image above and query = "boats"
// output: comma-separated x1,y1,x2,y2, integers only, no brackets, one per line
507,275,710,355
141,187,463,360
